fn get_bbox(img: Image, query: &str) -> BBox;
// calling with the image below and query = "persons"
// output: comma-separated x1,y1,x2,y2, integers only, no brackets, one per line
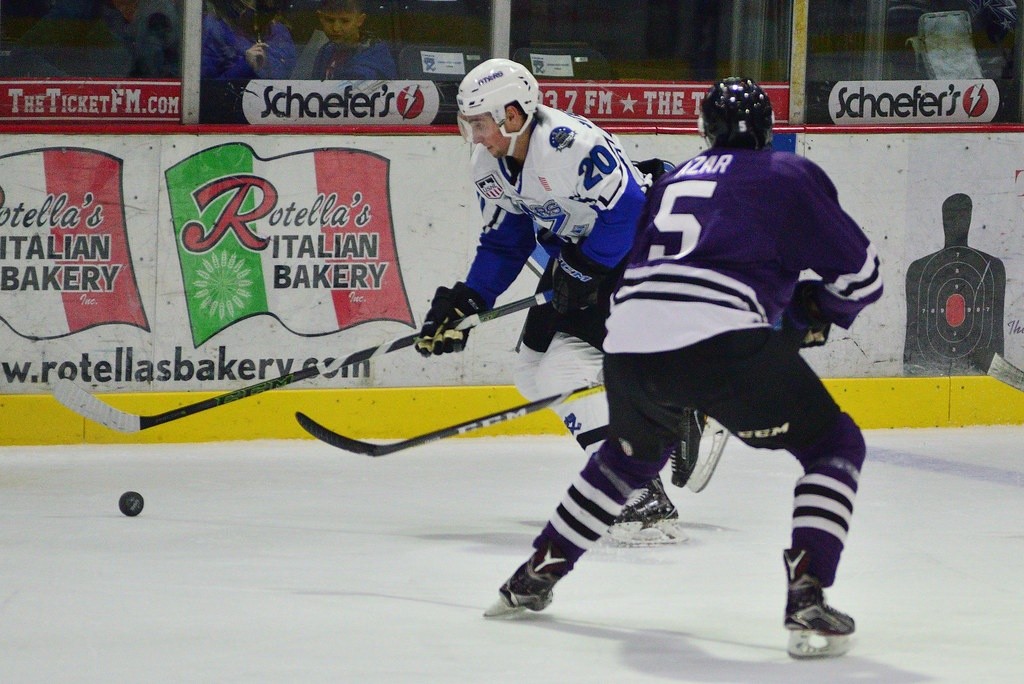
484,76,885,658
2,0,399,81
415,57,730,548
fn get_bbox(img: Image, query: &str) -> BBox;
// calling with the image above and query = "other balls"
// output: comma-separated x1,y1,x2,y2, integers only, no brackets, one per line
118,490,146,516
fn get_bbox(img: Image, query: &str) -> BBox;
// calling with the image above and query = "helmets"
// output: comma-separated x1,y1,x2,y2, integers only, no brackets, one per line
455,57,539,143
701,76,773,147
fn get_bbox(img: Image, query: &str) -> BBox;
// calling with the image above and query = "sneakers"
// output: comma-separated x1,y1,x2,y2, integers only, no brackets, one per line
482,548,574,619
784,546,855,658
672,408,729,490
609,479,689,547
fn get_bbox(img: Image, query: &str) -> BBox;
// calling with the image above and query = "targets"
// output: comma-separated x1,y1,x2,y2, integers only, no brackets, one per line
900,190,1008,378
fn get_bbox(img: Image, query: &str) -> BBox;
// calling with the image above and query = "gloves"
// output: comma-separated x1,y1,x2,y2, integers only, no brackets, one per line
551,241,600,314
780,281,831,346
417,282,482,355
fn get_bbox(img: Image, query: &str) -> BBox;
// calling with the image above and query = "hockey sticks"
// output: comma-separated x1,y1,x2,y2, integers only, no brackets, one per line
297,323,825,459
47,297,544,434
